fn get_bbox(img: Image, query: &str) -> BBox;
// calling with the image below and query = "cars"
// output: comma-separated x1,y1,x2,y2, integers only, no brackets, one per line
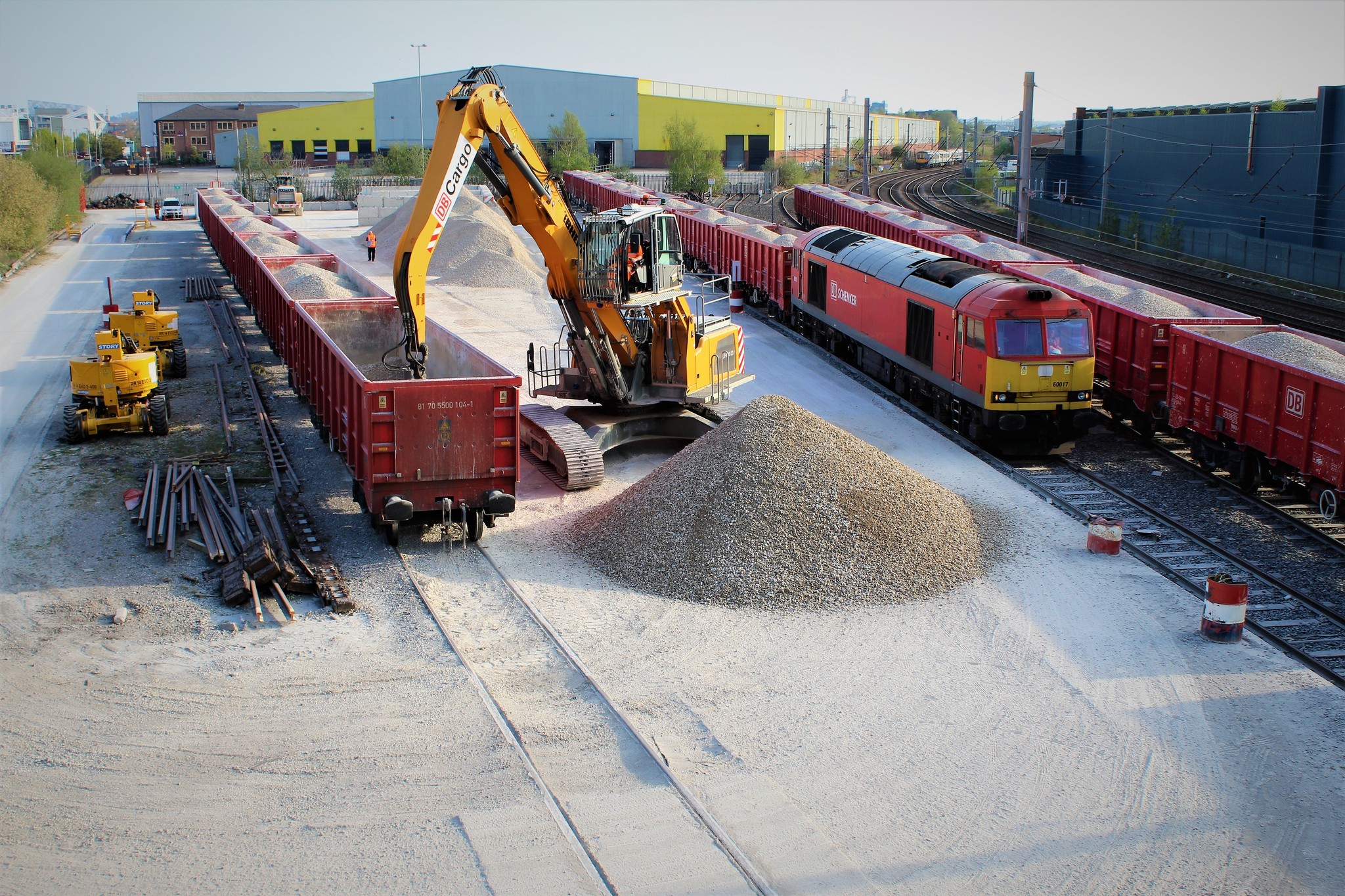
93,157,99,162
111,160,128,166
77,155,83,159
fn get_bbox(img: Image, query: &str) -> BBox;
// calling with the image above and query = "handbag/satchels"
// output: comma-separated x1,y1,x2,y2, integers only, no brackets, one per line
367,240,373,247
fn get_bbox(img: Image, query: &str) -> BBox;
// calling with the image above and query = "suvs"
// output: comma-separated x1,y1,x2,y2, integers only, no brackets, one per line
84,154,92,160
160,197,184,221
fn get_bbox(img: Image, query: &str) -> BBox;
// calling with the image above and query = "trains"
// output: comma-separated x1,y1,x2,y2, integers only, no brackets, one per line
794,182,1345,519
194,186,523,551
562,169,1097,458
915,149,968,169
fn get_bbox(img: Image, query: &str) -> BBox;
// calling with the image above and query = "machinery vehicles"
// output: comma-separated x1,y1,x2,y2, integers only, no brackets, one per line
372,66,773,479
108,290,188,379
266,175,304,216
61,328,171,439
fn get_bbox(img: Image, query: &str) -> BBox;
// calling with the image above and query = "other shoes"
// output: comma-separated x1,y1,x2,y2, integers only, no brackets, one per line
372,260,374,261
156,217,161,220
368,260,371,261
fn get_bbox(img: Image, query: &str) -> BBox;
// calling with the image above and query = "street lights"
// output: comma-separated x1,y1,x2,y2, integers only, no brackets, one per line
154,135,158,157
7,130,24,149
411,44,428,176
84,128,92,168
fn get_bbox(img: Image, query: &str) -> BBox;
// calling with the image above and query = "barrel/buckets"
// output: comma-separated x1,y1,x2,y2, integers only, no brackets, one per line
730,289,743,313
139,200,145,209
1087,516,1123,557
1200,573,1248,644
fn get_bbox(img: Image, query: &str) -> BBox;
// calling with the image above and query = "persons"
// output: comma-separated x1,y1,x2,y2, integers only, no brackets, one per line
1048,328,1062,354
683,189,696,201
283,178,289,184
366,231,376,262
607,239,643,295
155,200,161,220
